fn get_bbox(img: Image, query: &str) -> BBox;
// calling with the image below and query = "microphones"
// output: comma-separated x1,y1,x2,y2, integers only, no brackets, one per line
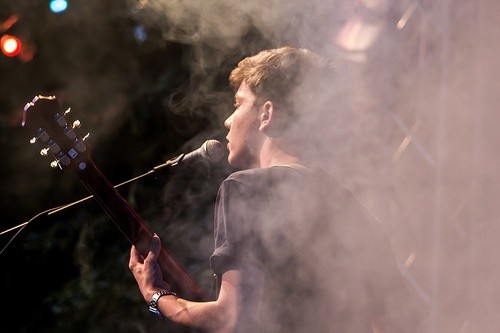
154,140,224,176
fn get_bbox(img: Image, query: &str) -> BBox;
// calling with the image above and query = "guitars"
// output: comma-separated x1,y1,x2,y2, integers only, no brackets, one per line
21,95,215,309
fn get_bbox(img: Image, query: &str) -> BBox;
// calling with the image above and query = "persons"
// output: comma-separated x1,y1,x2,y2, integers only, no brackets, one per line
126,46,361,333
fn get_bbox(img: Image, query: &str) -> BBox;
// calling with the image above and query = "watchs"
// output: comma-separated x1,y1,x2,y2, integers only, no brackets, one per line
148,288,178,321
